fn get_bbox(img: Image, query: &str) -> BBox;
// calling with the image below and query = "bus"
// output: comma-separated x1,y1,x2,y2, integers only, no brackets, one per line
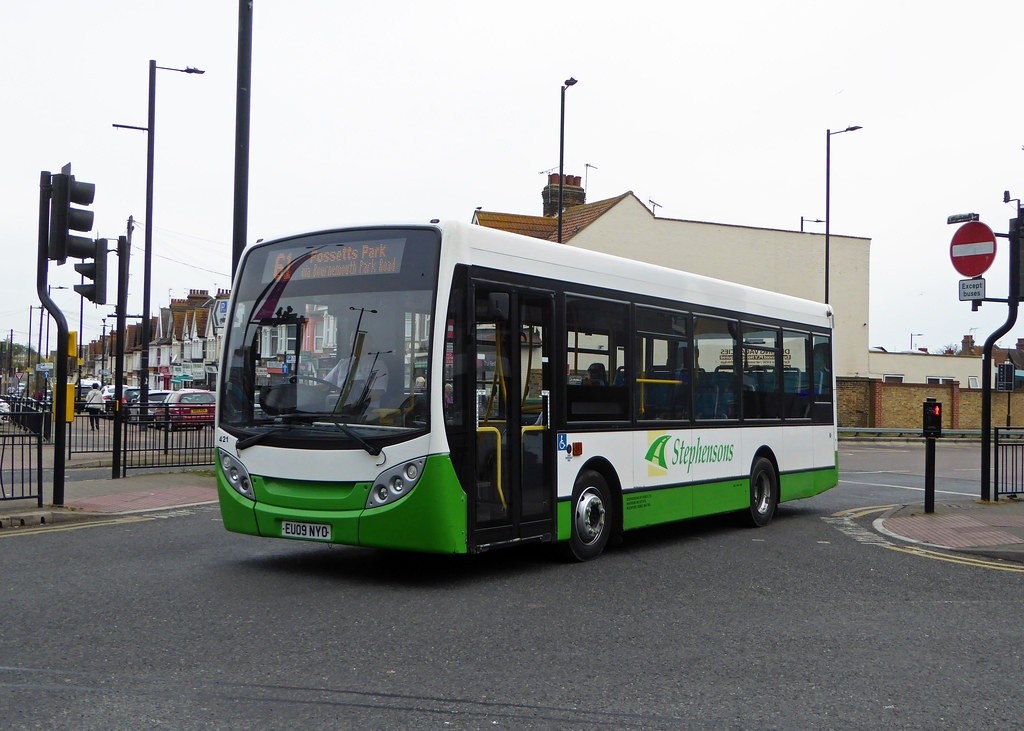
212,221,838,566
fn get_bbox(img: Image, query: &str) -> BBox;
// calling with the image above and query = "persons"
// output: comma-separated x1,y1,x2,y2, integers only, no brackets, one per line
413,376,425,388
582,362,610,387
318,326,390,425
7,383,16,395
85,383,106,431
445,383,454,409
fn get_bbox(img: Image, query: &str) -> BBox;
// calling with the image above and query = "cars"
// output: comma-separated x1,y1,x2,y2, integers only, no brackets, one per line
74,384,151,419
0,399,12,426
128,388,174,424
154,388,216,431
16,383,52,403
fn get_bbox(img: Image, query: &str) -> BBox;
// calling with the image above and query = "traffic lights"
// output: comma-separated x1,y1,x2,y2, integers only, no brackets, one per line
922,401,942,439
73,238,108,305
46,174,96,262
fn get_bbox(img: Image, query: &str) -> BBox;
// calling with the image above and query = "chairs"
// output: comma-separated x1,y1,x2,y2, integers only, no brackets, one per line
588,364,823,421
181,398,189,403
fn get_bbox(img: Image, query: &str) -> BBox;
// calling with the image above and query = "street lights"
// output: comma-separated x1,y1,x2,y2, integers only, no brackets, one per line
43,285,69,410
137,56,207,431
910,332,923,350
558,75,581,241
823,123,864,306
101,318,108,387
26,305,43,397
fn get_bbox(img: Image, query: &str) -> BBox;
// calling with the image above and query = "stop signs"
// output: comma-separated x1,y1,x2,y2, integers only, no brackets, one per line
948,221,998,279
